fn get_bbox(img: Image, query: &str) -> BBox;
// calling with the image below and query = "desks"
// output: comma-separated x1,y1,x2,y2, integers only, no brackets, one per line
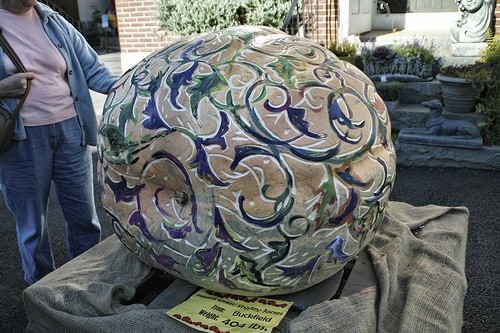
19,195,470,333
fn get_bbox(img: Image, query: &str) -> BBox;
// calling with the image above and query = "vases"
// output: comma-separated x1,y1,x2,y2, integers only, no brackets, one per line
436,71,493,113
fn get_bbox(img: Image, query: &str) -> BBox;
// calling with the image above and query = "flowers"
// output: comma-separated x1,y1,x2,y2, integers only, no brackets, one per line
437,59,497,100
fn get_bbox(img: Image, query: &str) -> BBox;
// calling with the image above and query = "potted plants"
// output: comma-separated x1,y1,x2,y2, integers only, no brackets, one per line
329,39,357,65
376,79,404,109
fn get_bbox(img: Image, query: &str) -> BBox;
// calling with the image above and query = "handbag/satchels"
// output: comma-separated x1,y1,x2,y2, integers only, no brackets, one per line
0,106,15,150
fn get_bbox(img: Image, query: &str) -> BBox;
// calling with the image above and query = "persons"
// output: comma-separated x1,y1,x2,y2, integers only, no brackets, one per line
451,0,497,43
1,0,120,285
